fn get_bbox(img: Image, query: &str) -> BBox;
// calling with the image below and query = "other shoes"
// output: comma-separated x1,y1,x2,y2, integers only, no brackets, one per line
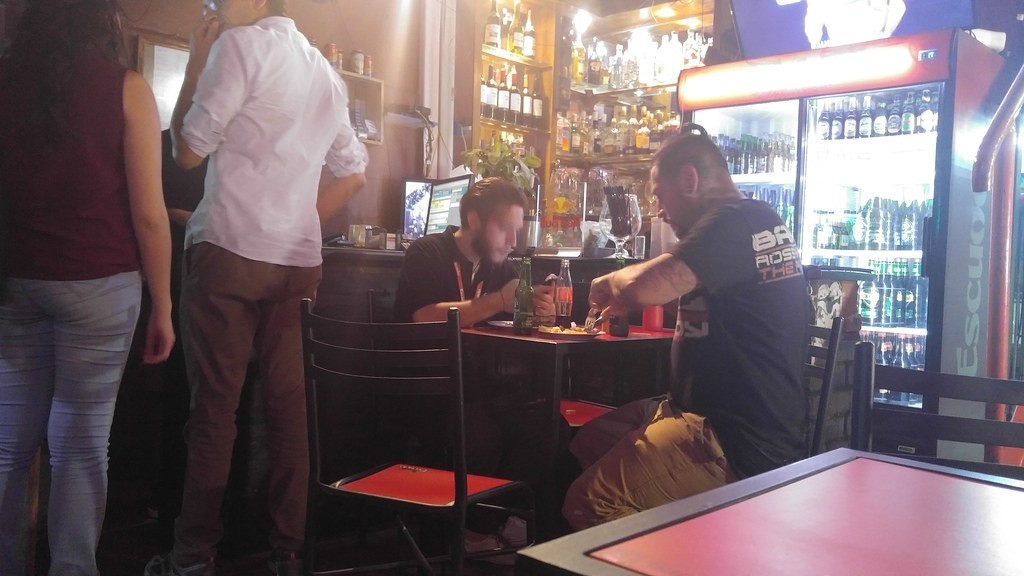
266,549,303,576
145,555,215,576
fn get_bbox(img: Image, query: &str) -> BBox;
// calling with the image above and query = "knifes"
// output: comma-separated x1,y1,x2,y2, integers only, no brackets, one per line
584,316,602,330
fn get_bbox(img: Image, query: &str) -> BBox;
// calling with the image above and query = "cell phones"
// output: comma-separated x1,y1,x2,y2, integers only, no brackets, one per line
542,277,558,285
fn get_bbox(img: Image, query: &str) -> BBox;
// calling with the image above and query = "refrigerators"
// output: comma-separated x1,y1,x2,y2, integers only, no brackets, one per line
678,27,1008,458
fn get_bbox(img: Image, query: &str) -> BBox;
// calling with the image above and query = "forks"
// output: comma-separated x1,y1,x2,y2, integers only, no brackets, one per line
585,305,595,332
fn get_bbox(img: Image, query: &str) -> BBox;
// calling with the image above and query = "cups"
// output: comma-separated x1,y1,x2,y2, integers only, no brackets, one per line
348,225,365,247
634,236,645,258
643,306,663,331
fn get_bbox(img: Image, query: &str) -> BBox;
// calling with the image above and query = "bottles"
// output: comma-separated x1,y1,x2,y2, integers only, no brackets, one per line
309,38,316,46
609,256,629,337
554,259,573,328
860,329,927,406
556,104,681,156
338,49,343,70
708,132,796,174
732,186,934,324
365,225,372,240
817,89,939,140
574,29,714,90
484,0,536,58
514,257,533,336
480,64,543,128
365,54,373,77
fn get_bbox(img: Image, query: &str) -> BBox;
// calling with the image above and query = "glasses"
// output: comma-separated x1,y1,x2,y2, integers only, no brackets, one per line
201,0,226,18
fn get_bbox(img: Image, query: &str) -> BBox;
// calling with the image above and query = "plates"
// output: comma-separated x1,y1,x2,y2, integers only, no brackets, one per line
489,320,539,329
536,328,604,339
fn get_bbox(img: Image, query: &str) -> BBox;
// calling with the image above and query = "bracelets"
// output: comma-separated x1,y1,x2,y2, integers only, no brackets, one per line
500,292,504,312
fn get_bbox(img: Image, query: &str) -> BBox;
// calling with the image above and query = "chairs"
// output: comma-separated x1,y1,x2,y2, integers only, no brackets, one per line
298,289,1024,576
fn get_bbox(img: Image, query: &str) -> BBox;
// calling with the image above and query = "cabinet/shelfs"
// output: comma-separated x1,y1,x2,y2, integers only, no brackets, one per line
479,44,550,137
333,69,384,146
577,81,678,178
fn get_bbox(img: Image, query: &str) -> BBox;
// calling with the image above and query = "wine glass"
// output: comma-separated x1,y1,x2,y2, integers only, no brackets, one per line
599,194,642,258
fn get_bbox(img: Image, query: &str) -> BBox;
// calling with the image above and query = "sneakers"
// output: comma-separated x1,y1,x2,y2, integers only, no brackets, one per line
463,515,529,565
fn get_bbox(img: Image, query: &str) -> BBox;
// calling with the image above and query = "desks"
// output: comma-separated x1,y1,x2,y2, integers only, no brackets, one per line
465,322,673,493
518,447,1024,576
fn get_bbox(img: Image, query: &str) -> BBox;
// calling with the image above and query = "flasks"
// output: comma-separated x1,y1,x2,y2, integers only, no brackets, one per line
523,215,540,257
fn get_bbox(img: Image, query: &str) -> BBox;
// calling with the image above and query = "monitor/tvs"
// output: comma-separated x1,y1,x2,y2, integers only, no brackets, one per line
402,174,474,241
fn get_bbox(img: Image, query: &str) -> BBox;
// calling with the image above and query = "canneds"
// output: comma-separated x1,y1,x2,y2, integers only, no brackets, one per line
323,43,373,78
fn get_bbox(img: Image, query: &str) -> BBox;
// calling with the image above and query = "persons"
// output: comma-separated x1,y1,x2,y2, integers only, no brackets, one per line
0,0,176,576
144,0,370,576
395,177,559,564
562,131,816,530
161,130,209,250
